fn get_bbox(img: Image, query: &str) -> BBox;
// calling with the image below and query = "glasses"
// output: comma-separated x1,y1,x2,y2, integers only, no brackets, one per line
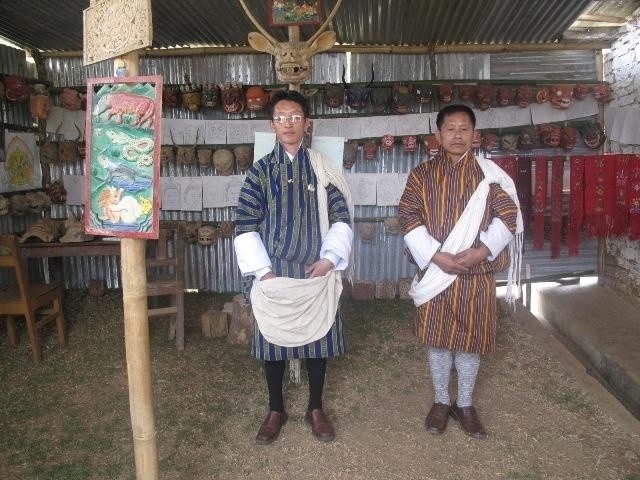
271,115,305,124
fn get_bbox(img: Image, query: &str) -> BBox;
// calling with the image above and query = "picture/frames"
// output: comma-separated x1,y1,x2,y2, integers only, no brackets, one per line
82,0,155,67
83,75,163,241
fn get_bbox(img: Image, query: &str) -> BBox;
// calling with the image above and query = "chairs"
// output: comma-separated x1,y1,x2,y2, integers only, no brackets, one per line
1,232,68,364
144,219,187,352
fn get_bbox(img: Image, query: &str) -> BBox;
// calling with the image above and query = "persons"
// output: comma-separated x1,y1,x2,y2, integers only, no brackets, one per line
398,106,525,436
234,91,355,446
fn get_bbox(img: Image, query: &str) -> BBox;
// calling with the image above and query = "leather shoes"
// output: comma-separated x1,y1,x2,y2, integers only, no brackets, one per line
424,403,451,434
255,410,289,444
305,407,335,441
450,400,487,438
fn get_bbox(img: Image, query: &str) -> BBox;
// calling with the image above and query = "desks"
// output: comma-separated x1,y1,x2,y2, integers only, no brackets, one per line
15,239,121,258
